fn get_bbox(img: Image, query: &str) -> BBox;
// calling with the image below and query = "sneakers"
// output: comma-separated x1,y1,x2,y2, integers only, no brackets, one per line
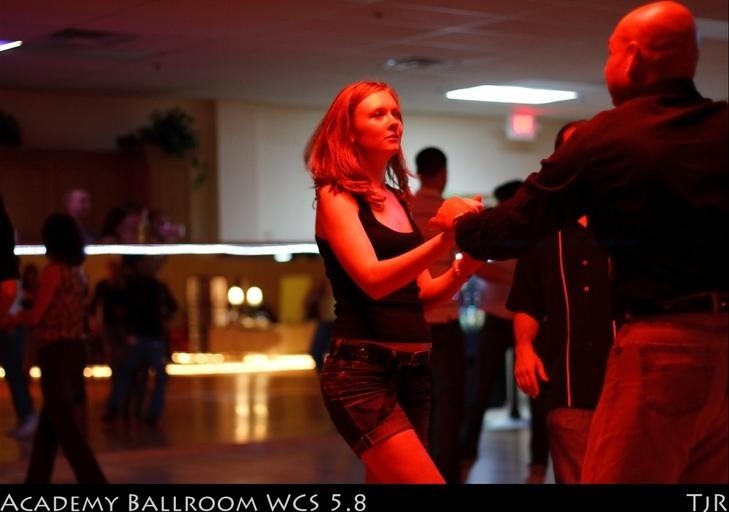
5,413,40,440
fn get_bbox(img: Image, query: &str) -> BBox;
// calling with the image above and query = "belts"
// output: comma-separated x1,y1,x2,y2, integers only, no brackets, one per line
329,341,435,369
616,292,728,319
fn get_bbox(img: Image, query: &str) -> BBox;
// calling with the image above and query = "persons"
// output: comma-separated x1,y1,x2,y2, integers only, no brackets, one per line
429,0,729,484
302,82,486,484
